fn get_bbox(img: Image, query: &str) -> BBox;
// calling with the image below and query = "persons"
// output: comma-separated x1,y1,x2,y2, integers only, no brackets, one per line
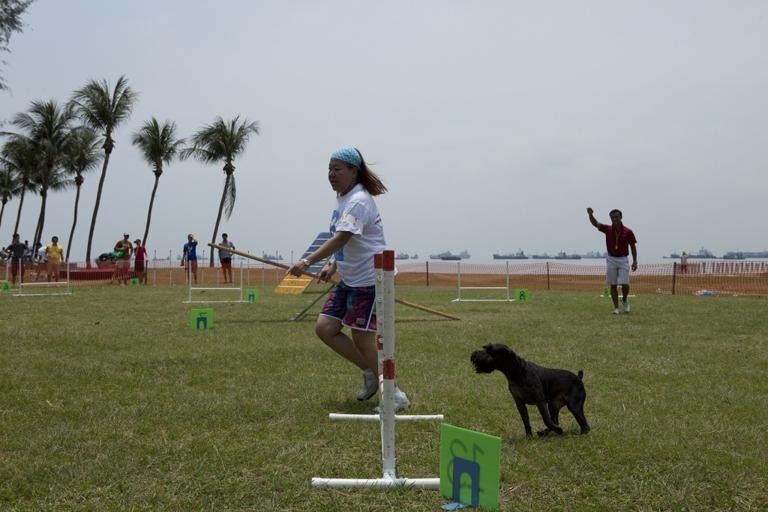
3,233,29,289
45,236,64,282
113,232,133,285
681,251,689,273
180,234,198,284
586,207,637,314
0,239,47,281
133,239,147,283
219,233,235,283
285,144,411,413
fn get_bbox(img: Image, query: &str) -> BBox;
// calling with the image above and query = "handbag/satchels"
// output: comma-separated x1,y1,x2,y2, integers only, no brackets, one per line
112,251,124,258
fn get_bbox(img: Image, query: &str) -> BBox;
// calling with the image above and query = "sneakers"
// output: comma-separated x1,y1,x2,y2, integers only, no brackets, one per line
376,392,410,414
357,368,378,401
610,296,630,314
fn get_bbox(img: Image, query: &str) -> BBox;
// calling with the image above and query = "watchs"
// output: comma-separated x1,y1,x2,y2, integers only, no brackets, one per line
301,259,311,267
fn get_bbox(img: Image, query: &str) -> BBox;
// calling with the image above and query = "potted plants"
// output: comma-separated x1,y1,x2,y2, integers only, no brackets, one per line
94,252,119,270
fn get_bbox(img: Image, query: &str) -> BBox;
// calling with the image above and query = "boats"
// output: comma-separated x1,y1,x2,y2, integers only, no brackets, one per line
151,246,284,260
394,248,608,262
662,244,768,261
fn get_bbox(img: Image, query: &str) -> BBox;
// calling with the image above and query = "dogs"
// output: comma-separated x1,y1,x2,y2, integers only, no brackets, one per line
469,342,591,436
